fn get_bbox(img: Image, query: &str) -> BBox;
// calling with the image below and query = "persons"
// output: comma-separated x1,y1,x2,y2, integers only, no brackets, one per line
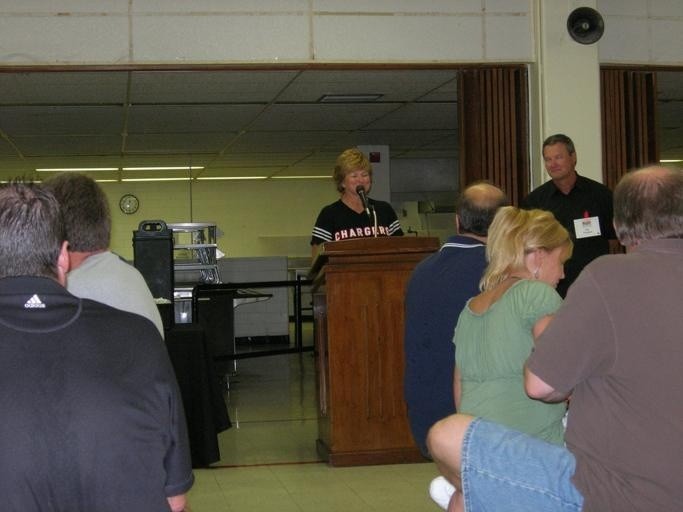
311,146,402,262
450,206,572,450
403,184,509,465
519,134,623,300
425,164,683,512
42,172,165,341
1,175,195,512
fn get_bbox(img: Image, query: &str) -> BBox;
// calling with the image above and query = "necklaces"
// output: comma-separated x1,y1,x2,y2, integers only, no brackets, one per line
507,275,521,279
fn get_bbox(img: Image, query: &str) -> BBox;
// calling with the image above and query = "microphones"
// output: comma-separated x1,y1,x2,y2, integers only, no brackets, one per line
357,185,372,217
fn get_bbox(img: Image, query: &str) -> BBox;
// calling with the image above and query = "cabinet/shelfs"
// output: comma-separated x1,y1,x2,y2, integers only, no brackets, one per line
166,222,218,284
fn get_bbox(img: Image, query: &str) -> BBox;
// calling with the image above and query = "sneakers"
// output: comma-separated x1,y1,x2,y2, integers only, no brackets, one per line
428,475,456,511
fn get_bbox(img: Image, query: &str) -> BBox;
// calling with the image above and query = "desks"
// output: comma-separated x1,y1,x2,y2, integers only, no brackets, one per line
163,323,233,474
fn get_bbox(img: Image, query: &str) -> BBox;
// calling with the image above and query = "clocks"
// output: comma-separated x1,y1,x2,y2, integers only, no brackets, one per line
121,192,141,215
